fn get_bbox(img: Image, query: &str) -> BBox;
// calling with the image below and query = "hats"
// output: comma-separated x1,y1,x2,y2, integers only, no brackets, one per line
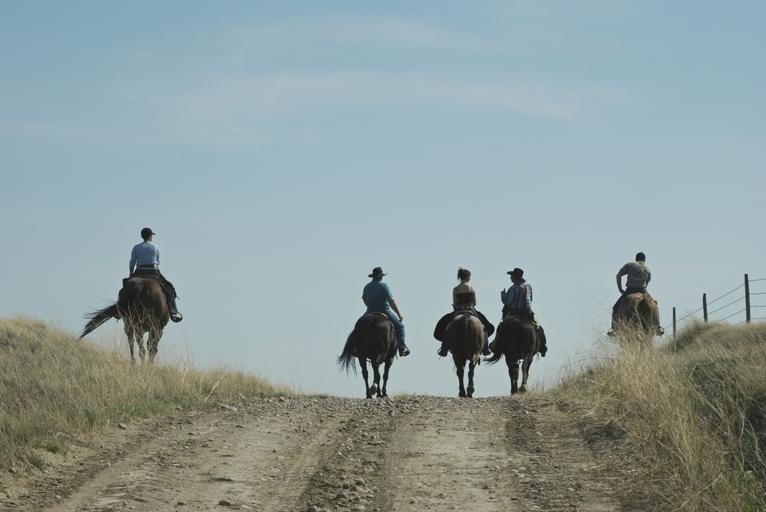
141,228,156,235
506,268,525,282
368,267,387,277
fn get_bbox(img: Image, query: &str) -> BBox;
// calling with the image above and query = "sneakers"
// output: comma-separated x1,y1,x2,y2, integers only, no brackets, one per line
653,328,664,335
482,349,491,356
438,347,447,356
607,331,618,337
170,313,182,322
399,348,409,356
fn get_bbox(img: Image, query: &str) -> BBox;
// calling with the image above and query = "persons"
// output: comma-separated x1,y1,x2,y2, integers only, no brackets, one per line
607,250,664,337
117,228,182,323
361,266,410,357
434,266,492,356
489,267,547,358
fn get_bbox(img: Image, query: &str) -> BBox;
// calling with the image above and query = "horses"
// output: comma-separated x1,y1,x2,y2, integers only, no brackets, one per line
482,318,540,396
336,311,398,399
78,276,170,367
444,313,485,398
616,293,656,358
